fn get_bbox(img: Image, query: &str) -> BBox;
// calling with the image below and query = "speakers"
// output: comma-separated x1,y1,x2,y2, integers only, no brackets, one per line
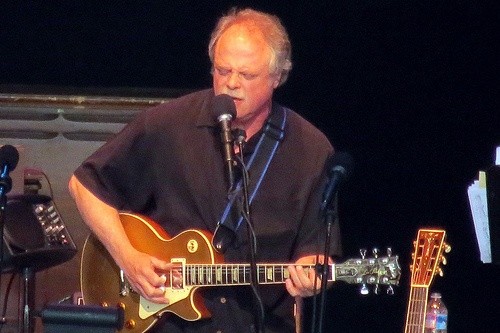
0,194,78,275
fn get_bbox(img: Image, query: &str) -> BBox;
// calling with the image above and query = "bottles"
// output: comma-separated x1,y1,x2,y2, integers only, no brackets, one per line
425,293,448,333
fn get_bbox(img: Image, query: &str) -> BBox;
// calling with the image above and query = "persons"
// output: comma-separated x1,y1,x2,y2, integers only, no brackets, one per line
67,7,344,333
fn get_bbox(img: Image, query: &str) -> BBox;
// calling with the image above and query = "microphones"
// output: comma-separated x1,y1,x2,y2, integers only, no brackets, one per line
0,145,19,175
212,94,237,188
318,153,355,221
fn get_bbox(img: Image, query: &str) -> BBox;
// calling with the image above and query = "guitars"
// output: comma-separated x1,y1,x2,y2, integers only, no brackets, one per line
79,211,401,333
404,228,451,333
